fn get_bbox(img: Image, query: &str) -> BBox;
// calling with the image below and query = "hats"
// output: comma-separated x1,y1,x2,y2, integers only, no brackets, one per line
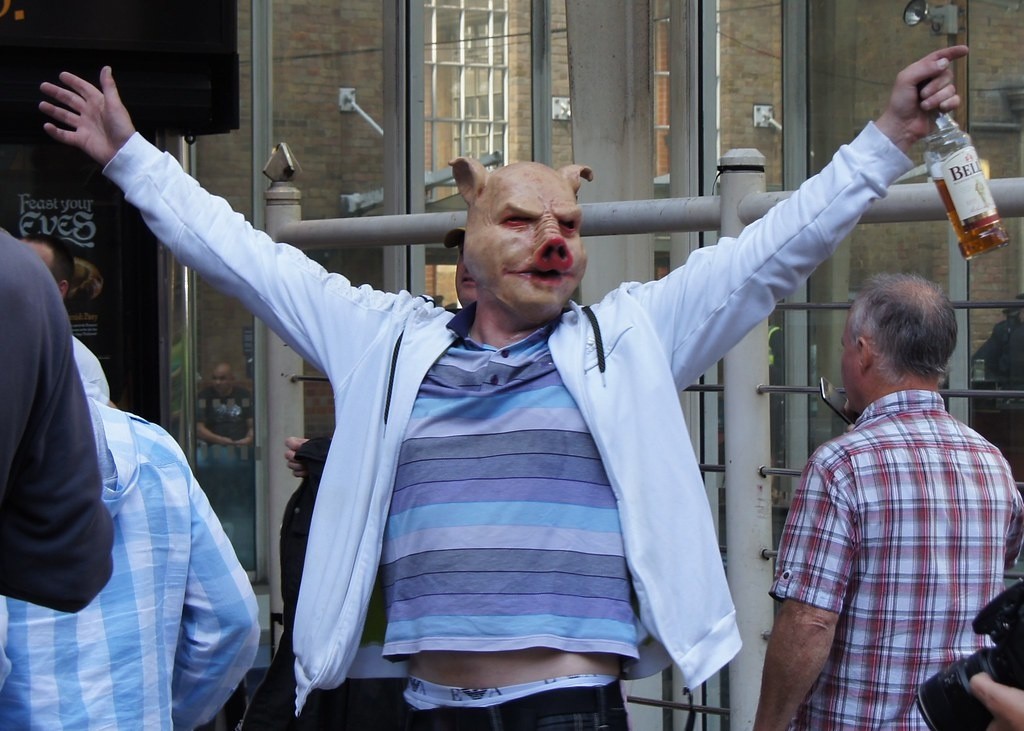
440,225,471,247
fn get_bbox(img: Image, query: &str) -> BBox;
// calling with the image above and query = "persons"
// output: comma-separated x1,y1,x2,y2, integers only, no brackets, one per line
197,350,257,531
38,42,976,731
12,238,115,419
0,228,115,624
744,266,1023,731
963,666,1023,731
0,393,265,728
265,220,482,710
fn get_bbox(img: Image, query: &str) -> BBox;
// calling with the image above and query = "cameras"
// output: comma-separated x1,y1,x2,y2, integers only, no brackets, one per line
915,581,1024,731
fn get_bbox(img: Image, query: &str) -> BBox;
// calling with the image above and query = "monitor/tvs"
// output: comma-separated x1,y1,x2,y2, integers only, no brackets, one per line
0,1,238,54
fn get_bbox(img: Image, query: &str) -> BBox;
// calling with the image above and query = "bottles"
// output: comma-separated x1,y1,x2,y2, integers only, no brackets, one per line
919,76,1012,261
